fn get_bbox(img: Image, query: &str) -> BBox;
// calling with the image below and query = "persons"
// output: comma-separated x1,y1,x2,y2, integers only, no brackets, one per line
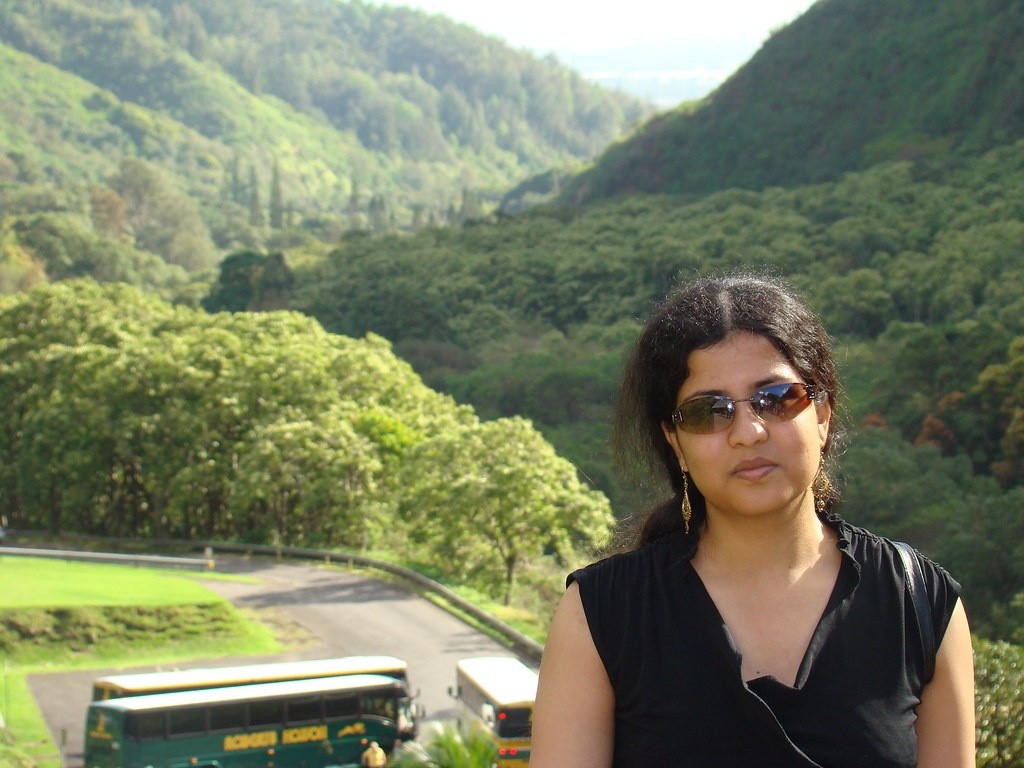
532,276,978,768
361,738,405,768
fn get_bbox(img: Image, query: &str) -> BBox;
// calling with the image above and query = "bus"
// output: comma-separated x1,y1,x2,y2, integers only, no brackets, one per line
445,657,539,768
82,655,428,768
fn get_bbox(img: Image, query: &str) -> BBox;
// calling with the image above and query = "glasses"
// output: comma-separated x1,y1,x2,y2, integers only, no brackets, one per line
670,383,819,433
